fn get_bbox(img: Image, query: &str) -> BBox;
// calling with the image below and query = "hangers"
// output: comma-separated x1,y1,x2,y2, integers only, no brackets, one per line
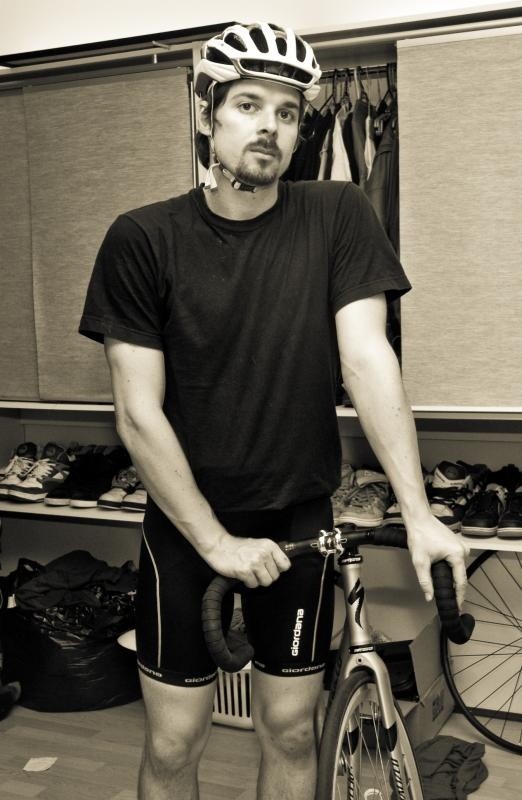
295,63,397,143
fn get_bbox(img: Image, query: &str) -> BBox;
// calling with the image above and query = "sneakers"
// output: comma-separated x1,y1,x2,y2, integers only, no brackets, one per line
338,470,390,527
44,444,104,505
330,462,356,525
122,481,148,512
497,471,522,537
70,444,133,507
97,464,140,509
460,463,519,536
0,441,38,496
9,442,74,502
429,460,480,533
383,472,433,527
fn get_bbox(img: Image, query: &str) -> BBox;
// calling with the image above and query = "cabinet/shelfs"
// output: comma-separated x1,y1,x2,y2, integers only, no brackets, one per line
1,401,522,557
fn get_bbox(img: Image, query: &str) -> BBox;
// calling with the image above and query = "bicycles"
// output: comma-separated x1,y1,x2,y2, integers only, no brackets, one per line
201,527,477,800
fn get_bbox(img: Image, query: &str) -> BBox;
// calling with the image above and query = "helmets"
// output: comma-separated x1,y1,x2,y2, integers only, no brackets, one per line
192,22,323,106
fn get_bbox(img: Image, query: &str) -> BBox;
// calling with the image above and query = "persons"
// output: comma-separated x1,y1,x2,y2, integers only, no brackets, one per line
78,21,470,800
